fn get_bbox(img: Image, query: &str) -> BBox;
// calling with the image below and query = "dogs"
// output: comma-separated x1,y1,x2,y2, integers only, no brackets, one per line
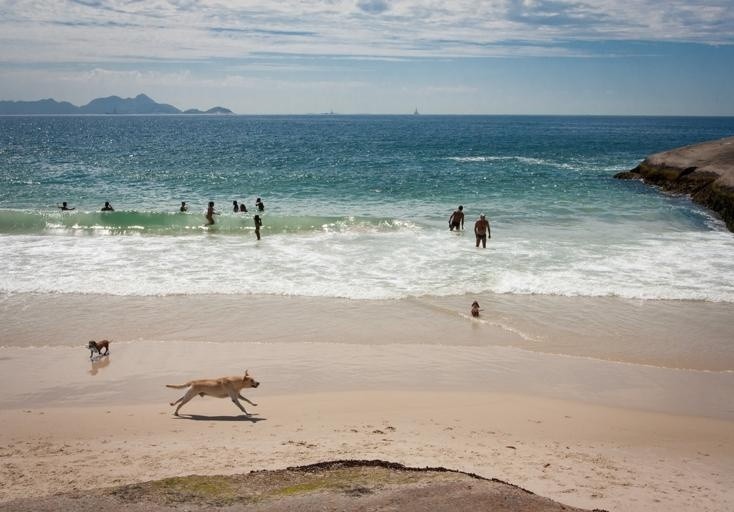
471,300,481,318
164,367,261,416
86,339,113,358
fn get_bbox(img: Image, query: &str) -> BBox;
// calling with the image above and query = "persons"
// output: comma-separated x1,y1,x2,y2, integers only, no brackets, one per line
448,206,464,231
254,215,262,240
181,202,188,211
474,213,491,248
233,198,264,212
57,202,76,210
205,202,220,225
102,202,114,210
